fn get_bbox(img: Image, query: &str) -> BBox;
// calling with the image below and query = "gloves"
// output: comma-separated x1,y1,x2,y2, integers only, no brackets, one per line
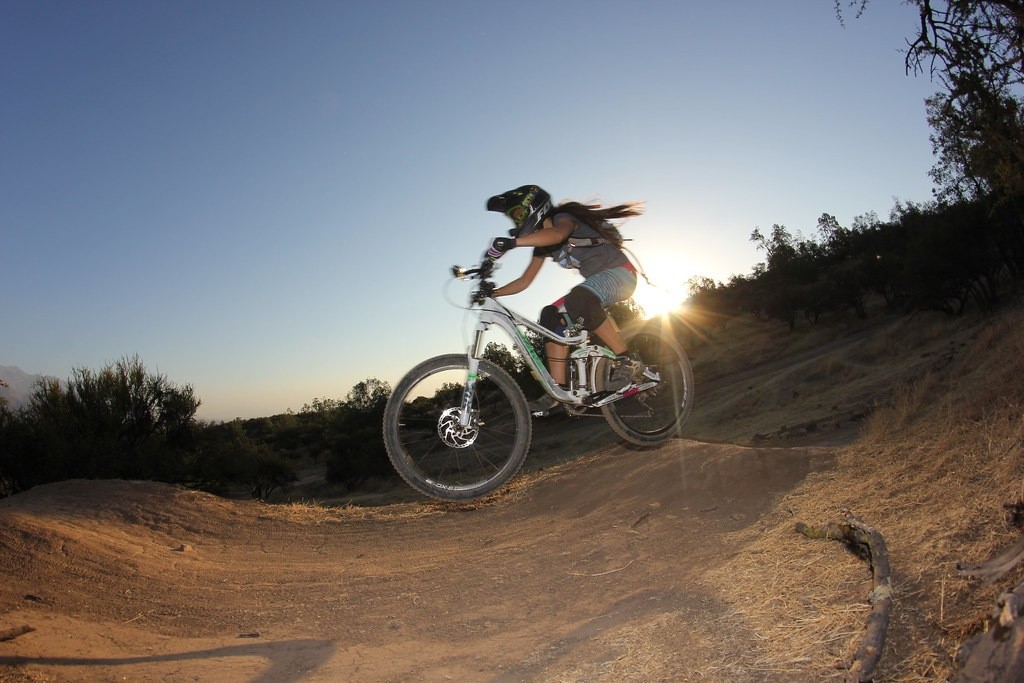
482,283,495,298
491,237,517,254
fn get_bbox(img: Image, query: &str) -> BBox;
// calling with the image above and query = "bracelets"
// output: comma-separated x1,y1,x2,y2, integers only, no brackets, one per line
493,288,498,297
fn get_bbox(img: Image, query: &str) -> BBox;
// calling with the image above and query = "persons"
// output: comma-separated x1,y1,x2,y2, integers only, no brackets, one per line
473,185,659,416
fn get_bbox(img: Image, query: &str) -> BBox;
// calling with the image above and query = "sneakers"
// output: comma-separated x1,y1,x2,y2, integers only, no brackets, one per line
608,353,645,392
527,386,571,412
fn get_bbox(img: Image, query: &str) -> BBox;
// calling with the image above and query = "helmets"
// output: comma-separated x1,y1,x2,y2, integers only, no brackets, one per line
487,184,552,239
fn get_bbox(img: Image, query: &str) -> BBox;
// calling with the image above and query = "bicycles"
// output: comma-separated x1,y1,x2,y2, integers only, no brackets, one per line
380,239,697,502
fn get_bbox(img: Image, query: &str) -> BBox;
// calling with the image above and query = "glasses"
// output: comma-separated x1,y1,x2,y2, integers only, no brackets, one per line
504,204,529,223
